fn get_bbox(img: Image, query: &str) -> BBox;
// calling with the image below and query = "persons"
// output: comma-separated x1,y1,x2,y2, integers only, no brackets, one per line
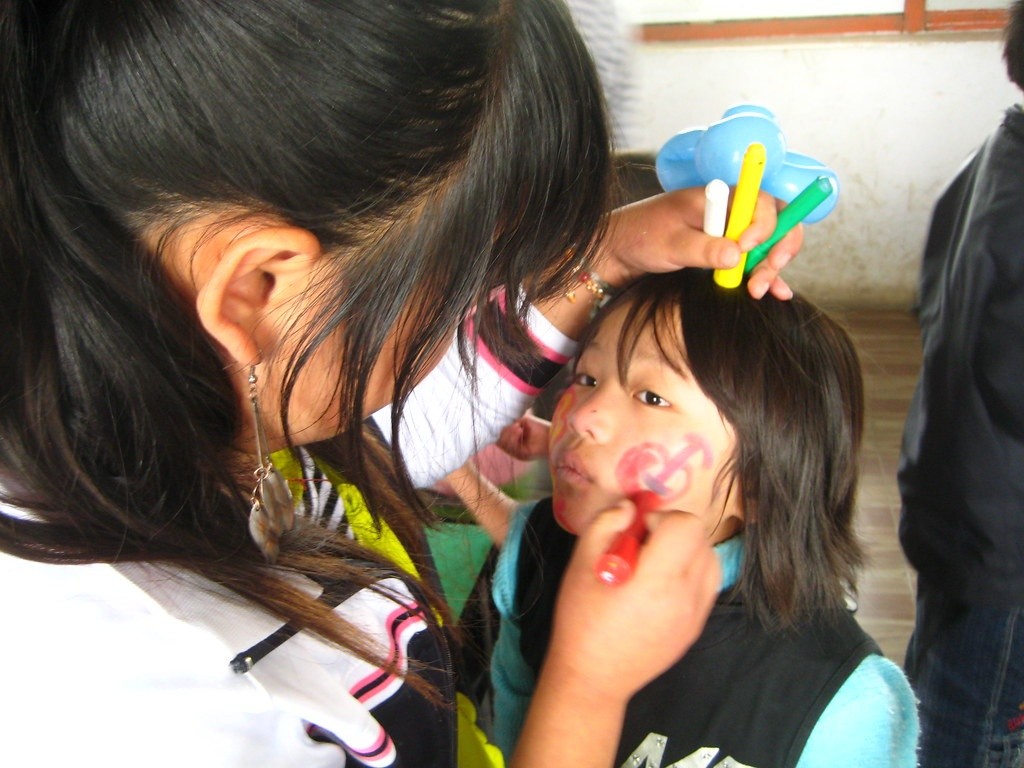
479,265,922,767
444,144,685,767
896,3,1024,768
0,1,803,767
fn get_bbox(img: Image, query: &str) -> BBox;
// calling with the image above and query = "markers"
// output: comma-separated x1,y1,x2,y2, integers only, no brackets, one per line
714,145,768,289
744,173,832,273
596,493,657,587
703,180,729,280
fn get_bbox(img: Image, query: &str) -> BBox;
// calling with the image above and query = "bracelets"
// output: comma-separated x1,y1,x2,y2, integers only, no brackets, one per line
560,248,620,311
